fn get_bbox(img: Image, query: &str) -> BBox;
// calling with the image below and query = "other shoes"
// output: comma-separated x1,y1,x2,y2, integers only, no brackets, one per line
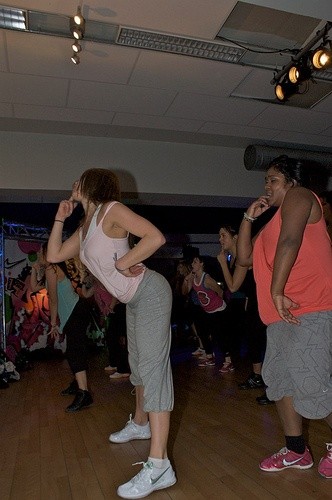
104,366,117,371
256,392,275,404
237,374,265,389
109,372,130,378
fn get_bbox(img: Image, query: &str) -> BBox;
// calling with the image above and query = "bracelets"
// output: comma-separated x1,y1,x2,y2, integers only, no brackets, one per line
243,213,257,222
55,220,64,223
51,326,56,328
116,267,125,271
185,279,188,281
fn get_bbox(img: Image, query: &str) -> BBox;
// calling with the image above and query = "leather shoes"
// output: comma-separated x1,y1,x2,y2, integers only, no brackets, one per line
61,378,79,396
64,389,94,412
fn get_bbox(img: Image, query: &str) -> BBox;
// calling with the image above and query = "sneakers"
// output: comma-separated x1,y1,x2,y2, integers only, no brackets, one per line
218,361,235,372
192,347,205,355
197,357,216,366
109,413,151,443
258,445,314,471
117,460,177,499
198,353,213,359
317,443,332,478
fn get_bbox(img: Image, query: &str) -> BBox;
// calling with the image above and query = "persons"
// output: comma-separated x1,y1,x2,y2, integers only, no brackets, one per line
68,264,131,379
177,256,233,372
31,244,94,413
46,168,177,499
319,191,332,241
236,154,332,478
217,224,268,390
